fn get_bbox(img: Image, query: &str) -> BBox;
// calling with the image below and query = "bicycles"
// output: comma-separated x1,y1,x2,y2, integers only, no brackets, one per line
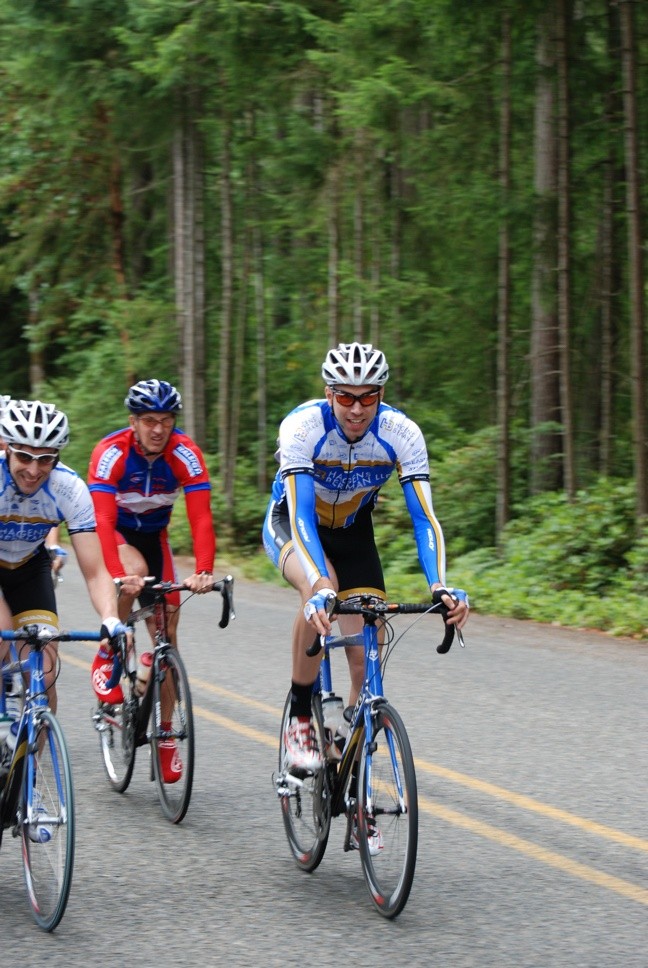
1,622,129,932
271,595,467,922
90,576,237,826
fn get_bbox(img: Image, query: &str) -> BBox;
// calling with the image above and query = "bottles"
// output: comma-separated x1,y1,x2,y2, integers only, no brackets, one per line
3,722,20,769
0,712,15,755
134,653,153,697
322,692,344,746
327,705,356,760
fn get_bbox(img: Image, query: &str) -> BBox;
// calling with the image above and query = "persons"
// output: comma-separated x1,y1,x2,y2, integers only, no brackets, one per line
262,342,472,858
0,398,134,842
87,378,217,783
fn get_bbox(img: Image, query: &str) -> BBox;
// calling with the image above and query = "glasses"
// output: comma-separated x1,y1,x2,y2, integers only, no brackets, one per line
10,446,58,466
136,416,175,427
330,389,381,407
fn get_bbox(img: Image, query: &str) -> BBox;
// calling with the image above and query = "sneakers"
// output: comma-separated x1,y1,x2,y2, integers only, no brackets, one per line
155,721,184,783
92,645,125,704
30,788,55,844
284,716,323,768
346,801,383,855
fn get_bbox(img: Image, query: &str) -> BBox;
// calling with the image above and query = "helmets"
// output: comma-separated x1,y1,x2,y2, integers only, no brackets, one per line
0,400,70,450
322,342,389,386
125,379,182,412
